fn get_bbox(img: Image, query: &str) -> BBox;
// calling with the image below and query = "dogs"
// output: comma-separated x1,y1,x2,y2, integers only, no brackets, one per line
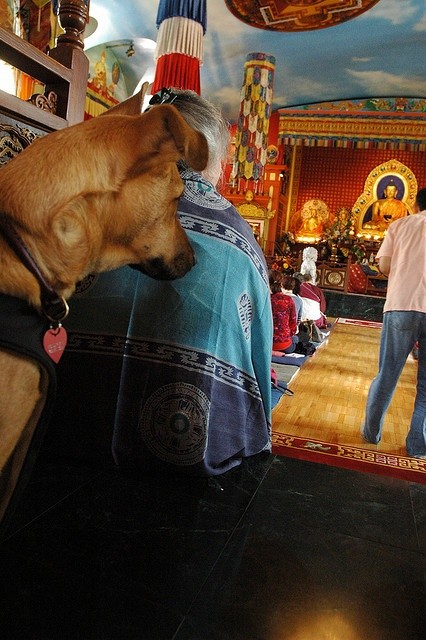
0,81,209,472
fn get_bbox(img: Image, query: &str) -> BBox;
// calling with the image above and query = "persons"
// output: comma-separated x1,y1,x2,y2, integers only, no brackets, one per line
268,268,327,353
303,208,321,236
57,88,272,479
362,186,426,455
362,181,408,238
